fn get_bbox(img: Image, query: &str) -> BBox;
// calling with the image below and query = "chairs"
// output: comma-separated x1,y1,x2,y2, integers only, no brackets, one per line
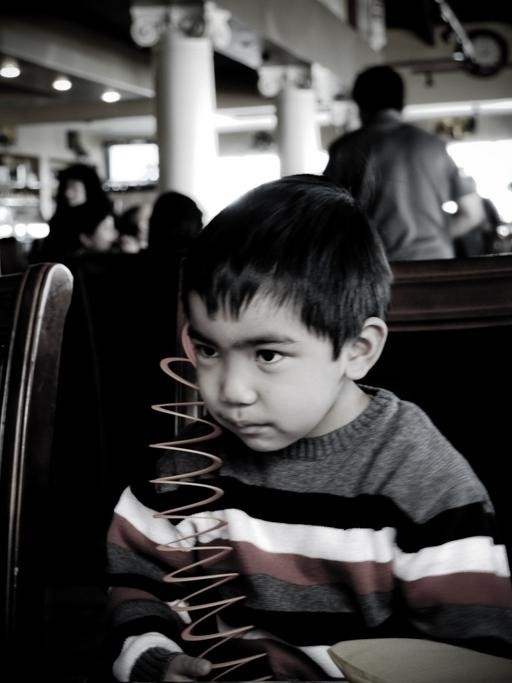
355,255,512,564
0,262,76,663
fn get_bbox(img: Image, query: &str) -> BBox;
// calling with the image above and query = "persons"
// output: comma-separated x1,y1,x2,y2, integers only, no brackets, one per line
457,172,500,256
104,171,511,683
324,62,484,259
32,161,201,263
